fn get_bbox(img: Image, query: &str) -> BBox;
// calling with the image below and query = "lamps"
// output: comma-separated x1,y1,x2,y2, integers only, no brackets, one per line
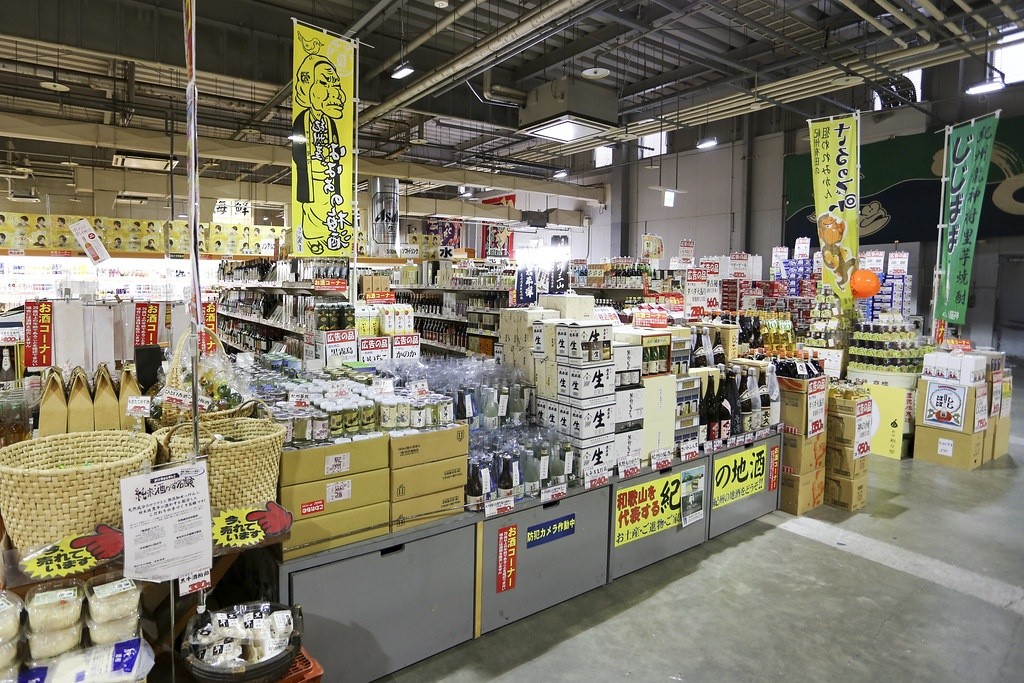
697,102,717,149
963,0,1005,95
390,0,415,80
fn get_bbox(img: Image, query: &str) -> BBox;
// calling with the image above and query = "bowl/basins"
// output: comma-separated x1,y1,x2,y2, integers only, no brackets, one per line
181,600,304,683
0,571,142,683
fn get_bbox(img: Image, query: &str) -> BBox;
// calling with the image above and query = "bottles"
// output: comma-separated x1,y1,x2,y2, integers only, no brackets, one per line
644,307,871,448
594,262,652,310
410,381,575,511
148,362,243,421
395,290,509,348
214,254,283,354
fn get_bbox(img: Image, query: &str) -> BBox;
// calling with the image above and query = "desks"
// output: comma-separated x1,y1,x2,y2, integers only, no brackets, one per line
0,528,292,661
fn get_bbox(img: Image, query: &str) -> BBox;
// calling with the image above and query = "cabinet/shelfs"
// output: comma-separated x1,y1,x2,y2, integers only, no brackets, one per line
568,282,665,323
390,284,508,353
217,282,349,358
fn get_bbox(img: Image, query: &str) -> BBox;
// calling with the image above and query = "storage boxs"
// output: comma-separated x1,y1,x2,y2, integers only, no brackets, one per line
317,277,414,338
0,570,143,683
469,260,1012,516
279,423,468,562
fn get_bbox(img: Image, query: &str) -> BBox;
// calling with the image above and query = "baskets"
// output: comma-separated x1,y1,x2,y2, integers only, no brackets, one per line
153,399,285,555
144,324,255,429
0,430,158,579
